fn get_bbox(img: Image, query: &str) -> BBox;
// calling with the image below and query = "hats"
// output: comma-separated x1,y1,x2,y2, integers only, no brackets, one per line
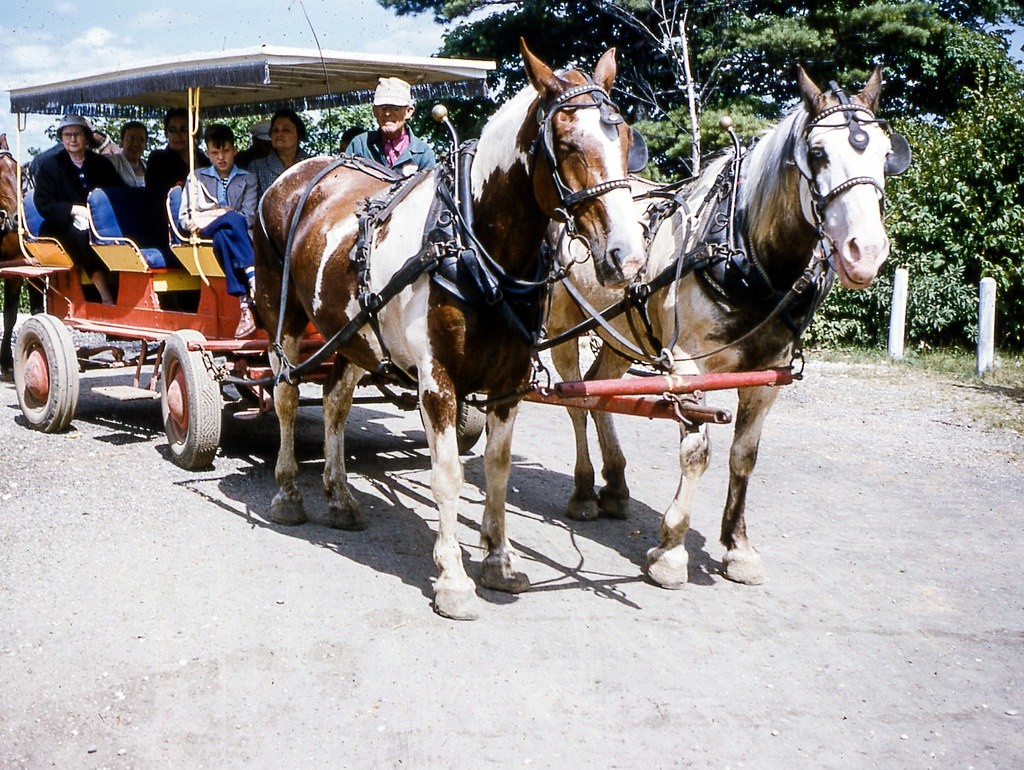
56,115,93,142
250,121,272,141
374,76,412,106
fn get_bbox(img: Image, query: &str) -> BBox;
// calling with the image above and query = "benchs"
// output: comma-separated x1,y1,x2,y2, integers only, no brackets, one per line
19,186,227,313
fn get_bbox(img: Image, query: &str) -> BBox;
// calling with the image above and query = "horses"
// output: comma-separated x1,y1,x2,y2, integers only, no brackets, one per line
247,31,648,621
549,61,914,596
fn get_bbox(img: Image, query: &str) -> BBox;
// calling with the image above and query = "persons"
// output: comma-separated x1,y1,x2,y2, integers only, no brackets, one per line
145,104,211,246
103,121,149,188
235,119,273,171
30,114,133,304
344,77,435,175
249,108,316,200
339,125,366,155
29,141,65,179
177,123,259,338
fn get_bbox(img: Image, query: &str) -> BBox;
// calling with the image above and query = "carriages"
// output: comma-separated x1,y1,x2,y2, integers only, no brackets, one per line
0,33,912,624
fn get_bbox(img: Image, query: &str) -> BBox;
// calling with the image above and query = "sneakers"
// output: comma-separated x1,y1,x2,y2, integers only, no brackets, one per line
235,303,257,338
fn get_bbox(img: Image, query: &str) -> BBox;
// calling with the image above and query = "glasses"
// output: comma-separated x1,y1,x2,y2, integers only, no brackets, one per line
167,125,187,133
62,132,85,137
